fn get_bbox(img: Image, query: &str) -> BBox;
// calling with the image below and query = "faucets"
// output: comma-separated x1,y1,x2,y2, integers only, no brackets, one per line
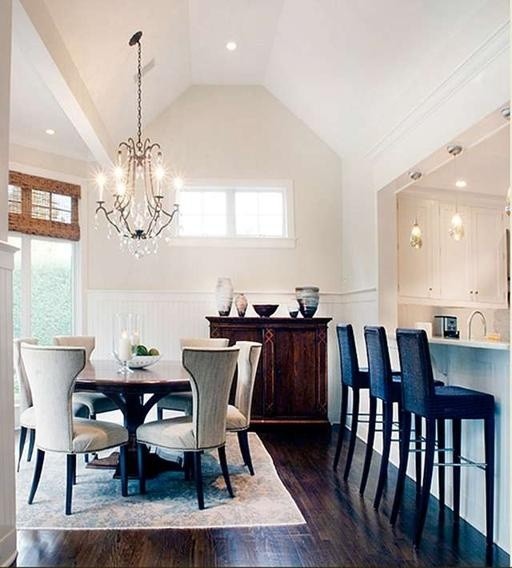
469,311,486,342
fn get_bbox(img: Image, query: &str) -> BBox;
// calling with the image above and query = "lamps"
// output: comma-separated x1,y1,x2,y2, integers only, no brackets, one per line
409,171,422,248
500,106,512,216
94,31,180,260
447,145,465,241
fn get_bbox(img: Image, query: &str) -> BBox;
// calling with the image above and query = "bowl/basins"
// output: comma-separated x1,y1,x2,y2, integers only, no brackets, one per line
111,355,163,369
252,304,279,318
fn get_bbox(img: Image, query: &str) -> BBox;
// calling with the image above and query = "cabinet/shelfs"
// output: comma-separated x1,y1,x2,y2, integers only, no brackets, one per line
205,317,332,431
439,189,508,309
398,186,440,306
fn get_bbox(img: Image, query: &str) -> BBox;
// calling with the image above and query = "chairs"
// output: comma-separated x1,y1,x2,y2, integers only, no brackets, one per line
20,342,129,515
157,338,230,420
390,329,495,549
359,326,444,508
13,337,90,472
53,335,126,455
333,323,401,481
191,341,263,475
136,345,240,510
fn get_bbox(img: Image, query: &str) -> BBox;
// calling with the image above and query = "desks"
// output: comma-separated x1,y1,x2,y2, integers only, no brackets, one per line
73,360,189,480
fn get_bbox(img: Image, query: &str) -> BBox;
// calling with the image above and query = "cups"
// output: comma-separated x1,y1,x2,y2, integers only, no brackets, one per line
287,307,299,318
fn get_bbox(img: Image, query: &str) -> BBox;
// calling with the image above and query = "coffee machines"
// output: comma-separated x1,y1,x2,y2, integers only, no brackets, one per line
433,315,457,337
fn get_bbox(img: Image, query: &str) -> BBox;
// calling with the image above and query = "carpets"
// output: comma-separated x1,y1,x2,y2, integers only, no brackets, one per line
14,432,307,530
